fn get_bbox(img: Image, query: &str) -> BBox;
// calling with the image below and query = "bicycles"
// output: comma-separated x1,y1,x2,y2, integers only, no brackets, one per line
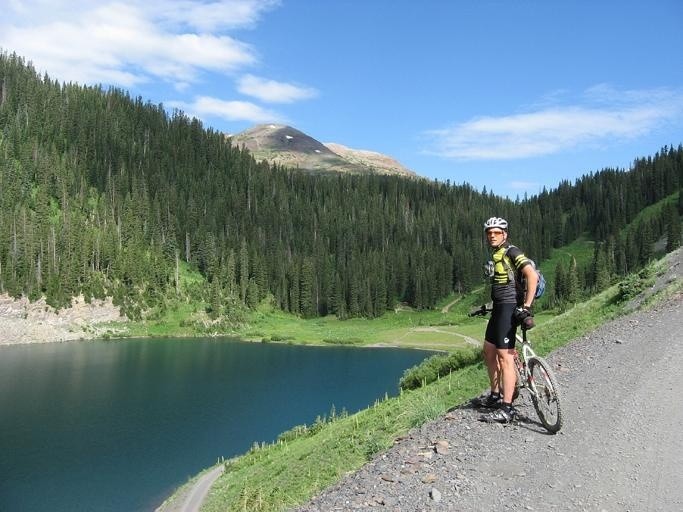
466,307,563,432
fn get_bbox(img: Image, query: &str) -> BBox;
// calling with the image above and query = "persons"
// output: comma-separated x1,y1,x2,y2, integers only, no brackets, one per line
469,217,541,424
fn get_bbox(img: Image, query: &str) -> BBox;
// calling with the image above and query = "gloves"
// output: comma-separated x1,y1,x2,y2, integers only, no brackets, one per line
514,306,531,319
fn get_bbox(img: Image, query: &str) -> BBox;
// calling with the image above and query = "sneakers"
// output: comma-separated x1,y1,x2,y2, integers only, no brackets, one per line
481,406,515,421
472,397,503,408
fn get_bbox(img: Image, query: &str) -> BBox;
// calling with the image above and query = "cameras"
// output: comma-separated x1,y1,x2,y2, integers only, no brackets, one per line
483,260,495,277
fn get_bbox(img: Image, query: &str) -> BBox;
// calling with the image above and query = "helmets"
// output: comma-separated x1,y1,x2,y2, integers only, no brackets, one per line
484,217,508,232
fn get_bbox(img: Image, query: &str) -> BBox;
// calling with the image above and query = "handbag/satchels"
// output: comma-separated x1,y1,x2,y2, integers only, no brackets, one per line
502,245,546,298
